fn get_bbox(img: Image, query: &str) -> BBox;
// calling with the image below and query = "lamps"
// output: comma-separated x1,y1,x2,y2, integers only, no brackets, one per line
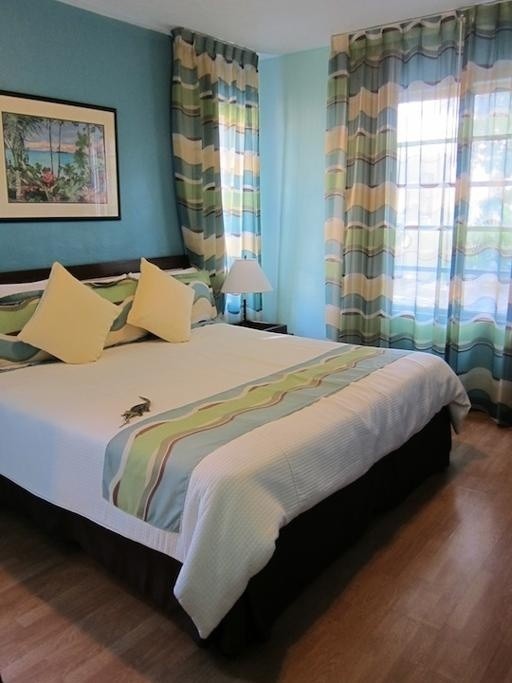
219,260,272,326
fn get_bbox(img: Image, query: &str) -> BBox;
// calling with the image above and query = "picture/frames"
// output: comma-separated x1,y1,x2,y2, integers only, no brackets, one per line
0,90,121,220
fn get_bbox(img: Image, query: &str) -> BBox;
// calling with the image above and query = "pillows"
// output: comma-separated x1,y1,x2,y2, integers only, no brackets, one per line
0,255,218,371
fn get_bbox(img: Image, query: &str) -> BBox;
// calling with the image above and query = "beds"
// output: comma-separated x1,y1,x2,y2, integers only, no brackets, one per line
0,256,449,642
